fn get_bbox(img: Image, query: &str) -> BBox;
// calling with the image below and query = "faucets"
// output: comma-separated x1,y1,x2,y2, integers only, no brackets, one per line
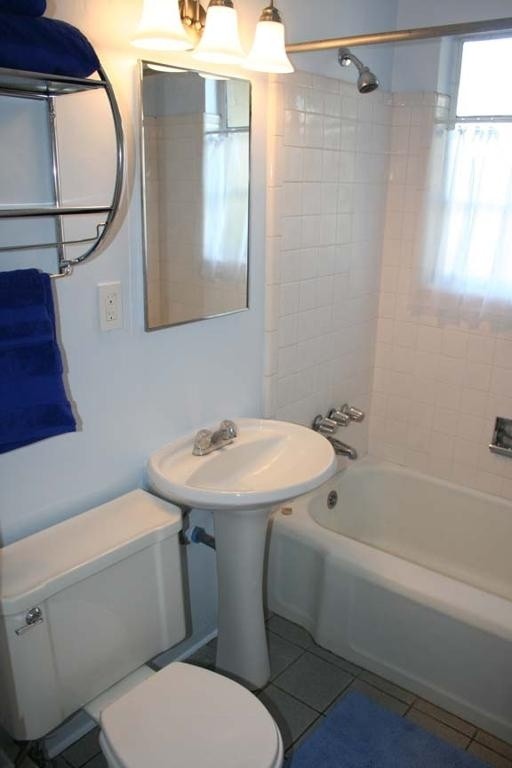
210,428,237,451
327,436,358,460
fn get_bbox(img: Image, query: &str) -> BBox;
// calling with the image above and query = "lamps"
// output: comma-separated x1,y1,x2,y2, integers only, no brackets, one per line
176,0,296,76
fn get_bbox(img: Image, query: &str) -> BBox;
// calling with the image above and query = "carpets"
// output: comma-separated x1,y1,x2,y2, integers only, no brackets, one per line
279,691,498,767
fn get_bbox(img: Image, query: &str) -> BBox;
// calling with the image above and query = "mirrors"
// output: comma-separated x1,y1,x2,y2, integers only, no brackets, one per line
137,58,254,332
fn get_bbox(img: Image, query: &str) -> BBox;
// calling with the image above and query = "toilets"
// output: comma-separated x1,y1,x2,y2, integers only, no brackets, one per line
0,487,286,768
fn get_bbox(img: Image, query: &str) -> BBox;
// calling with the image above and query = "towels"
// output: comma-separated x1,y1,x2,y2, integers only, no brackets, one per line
0,269,78,454
0,0,102,77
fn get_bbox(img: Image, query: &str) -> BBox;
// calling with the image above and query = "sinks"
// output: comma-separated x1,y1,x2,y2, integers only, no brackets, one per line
145,416,338,508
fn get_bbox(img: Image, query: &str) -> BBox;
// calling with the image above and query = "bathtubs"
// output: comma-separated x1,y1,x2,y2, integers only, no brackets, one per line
266,452,512,748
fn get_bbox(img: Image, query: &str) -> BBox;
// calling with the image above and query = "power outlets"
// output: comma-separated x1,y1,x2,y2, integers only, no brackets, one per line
98,281,126,331
